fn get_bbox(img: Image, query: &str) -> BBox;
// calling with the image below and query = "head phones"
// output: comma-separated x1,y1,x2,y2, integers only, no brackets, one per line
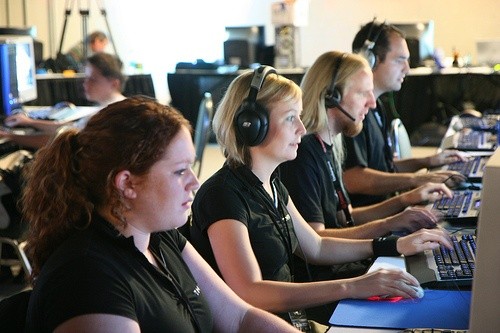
233,65,277,147
356,15,386,71
324,54,348,109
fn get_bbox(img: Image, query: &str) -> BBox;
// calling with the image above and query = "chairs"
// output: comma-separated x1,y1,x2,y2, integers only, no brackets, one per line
194,92,213,178
391,118,412,159
0,149,34,281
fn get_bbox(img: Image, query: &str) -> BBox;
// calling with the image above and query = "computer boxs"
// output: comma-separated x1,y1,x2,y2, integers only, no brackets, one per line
275,25,299,68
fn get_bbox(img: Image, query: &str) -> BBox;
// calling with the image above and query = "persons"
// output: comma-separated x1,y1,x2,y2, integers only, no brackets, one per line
63,30,107,73
19,94,311,333
339,20,470,194
188,64,455,324
275,50,454,278
0,52,127,150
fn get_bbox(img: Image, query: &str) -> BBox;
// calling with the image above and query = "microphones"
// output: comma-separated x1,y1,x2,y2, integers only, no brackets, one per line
336,105,356,122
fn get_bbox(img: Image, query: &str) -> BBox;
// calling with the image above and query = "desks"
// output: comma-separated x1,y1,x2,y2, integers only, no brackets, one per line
326,115,500,333
169,66,500,144
24,74,156,106
0,106,101,148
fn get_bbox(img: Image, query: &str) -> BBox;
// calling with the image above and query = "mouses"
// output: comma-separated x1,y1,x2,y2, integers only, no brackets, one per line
379,282,424,299
55,101,75,109
459,109,483,118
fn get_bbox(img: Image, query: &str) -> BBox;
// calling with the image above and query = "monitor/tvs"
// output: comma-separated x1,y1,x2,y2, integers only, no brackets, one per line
469,146,500,333
222,26,265,67
0,28,38,107
391,21,434,68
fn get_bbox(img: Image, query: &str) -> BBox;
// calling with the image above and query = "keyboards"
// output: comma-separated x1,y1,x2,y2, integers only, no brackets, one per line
429,156,489,181
403,328,469,333
29,107,71,121
442,118,494,150
412,190,482,223
404,233,477,288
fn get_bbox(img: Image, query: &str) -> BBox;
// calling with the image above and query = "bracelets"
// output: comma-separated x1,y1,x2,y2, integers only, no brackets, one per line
372,236,401,257
